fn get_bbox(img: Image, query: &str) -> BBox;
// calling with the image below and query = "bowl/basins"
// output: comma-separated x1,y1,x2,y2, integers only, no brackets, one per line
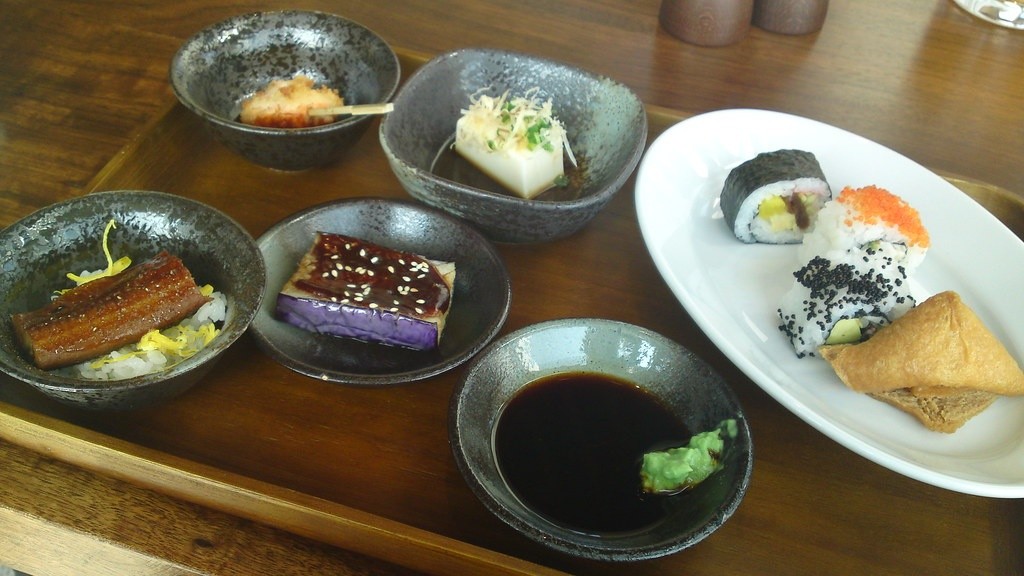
246,195,513,388
168,8,401,171
448,316,755,562
0,189,268,414
378,46,649,247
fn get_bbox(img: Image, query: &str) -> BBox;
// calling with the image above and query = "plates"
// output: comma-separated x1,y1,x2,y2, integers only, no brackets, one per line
633,106,1024,499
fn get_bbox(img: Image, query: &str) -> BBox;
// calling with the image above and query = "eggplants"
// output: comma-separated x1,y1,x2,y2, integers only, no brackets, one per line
276,231,458,351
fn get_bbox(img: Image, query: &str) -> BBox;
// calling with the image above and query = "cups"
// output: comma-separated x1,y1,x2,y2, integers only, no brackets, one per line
753,0,830,34
659,0,754,48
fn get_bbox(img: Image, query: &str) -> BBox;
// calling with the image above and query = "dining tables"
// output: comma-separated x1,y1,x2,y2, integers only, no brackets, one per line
0,0,1024,576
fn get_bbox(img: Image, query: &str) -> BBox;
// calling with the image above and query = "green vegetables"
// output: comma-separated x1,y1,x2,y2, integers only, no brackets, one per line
488,102,570,187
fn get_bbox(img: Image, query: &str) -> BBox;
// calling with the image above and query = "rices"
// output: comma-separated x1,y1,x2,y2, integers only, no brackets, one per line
56,268,229,380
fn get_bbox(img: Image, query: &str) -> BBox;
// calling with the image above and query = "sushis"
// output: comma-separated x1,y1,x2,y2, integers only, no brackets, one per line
719,149,930,357
